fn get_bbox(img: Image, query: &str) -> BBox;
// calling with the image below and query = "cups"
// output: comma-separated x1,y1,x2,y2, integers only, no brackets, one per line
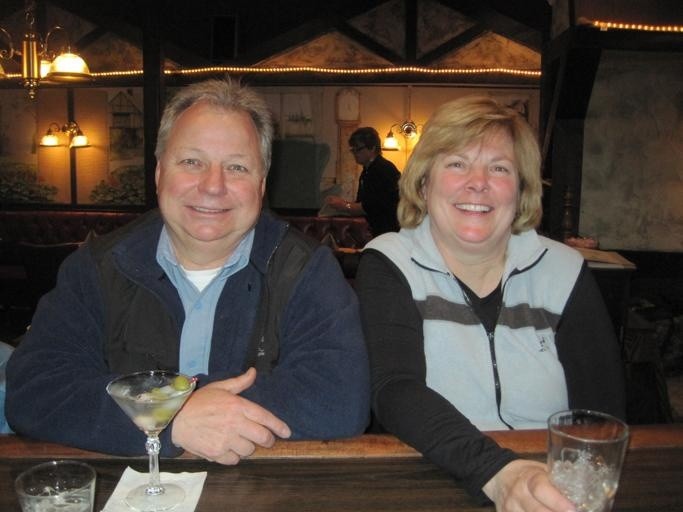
544,407,631,512
14,458,98,512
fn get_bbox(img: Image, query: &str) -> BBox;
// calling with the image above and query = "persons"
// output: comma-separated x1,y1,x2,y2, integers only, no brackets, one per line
327,125,405,234
348,94,633,512
0,76,373,468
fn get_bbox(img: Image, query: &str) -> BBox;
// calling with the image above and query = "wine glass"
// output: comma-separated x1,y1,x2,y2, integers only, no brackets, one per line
104,368,197,512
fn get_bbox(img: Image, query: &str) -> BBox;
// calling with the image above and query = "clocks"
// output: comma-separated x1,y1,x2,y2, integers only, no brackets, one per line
335,88,362,126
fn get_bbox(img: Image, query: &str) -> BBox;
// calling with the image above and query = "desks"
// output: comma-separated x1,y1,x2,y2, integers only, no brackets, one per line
579,250,637,363
0,422,683,512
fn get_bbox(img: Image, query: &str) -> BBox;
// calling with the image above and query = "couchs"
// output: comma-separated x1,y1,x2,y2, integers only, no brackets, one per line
304,217,373,288
0,202,146,328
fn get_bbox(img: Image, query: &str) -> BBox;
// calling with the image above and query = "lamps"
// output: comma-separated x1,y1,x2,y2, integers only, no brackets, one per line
38,120,91,150
381,122,424,152
0,1,98,98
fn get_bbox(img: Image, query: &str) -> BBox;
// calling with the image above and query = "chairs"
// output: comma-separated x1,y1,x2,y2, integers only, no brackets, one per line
15,241,86,298
